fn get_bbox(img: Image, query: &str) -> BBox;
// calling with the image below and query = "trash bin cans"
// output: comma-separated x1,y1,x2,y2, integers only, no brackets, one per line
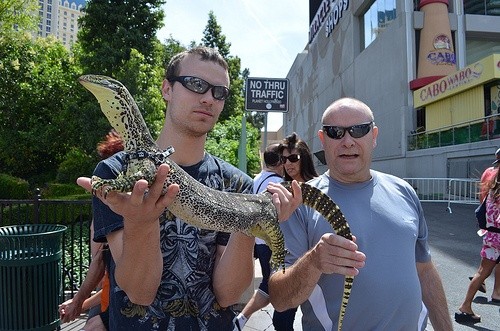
0,223,68,331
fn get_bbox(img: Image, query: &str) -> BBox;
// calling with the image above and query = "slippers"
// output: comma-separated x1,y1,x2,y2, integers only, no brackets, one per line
491,296,500,304
454,309,481,325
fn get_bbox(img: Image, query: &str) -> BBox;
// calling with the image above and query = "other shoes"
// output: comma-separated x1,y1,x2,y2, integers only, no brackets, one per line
469,275,487,294
236,312,249,329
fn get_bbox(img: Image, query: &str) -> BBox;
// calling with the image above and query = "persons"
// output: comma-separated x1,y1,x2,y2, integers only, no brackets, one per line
269,97,455,331
76,47,304,331
234,142,285,331
469,148,500,305
60,133,125,331
272,131,320,331
456,167,500,323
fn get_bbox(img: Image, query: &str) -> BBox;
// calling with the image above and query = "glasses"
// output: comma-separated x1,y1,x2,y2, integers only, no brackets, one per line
324,122,374,140
167,76,231,101
280,154,301,164
